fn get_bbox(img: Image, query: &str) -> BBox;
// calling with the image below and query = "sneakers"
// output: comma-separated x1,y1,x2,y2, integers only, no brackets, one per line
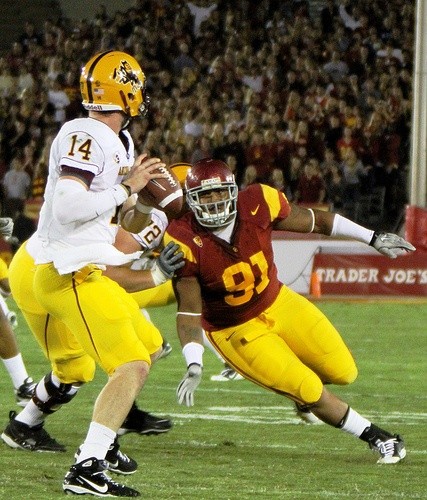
1,420,65,453
116,407,171,436
376,434,407,465
13,376,61,411
210,368,245,381
63,456,140,498
293,400,318,423
74,434,137,474
155,339,172,360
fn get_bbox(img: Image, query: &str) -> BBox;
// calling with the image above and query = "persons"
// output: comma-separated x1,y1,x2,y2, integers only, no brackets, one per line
0,218,37,404
0,151,186,453
161,160,417,462
0,0,416,217
36,50,167,500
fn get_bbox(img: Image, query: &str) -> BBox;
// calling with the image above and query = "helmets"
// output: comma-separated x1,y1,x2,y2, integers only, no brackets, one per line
80,50,146,116
166,163,193,188
186,159,234,224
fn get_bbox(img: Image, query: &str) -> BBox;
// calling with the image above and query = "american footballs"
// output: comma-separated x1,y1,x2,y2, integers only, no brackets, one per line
141,153,184,214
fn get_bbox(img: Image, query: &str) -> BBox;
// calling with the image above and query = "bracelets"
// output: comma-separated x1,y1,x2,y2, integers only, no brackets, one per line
121,182,130,196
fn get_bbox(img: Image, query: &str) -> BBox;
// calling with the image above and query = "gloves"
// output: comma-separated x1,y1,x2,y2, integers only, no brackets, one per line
177,364,202,407
156,241,185,278
369,231,416,258
